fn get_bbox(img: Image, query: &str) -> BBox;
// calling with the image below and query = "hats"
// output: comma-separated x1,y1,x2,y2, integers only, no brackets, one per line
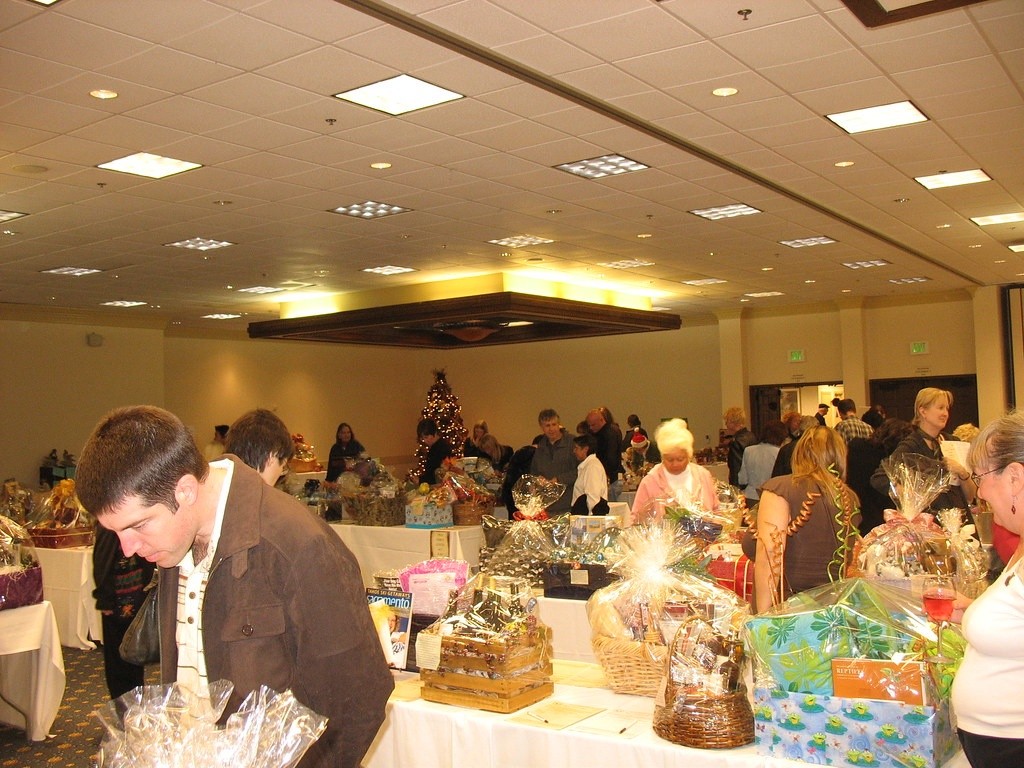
631,427,648,448
819,404,829,408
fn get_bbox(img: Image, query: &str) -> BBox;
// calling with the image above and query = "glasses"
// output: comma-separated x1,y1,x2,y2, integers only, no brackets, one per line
970,461,1024,488
280,457,291,476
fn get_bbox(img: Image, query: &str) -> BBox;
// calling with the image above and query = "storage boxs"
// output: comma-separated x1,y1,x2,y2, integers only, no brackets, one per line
405,505,453,529
753,686,963,768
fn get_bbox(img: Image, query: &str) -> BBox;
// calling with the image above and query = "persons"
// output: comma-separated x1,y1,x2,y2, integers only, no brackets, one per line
324,423,365,521
93,408,297,732
918,407,1024,768
741,425,860,615
411,407,662,520
73,406,395,768
630,418,721,525
722,388,1021,582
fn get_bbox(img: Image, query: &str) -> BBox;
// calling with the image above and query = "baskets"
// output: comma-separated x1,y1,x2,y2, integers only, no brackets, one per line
590,633,670,699
653,616,755,749
286,457,317,473
454,502,494,524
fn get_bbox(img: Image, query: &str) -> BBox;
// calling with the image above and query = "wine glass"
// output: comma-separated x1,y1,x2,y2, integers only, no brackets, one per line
923,577,957,664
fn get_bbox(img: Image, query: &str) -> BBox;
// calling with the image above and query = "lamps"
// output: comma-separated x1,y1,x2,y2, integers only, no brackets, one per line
247,265,684,349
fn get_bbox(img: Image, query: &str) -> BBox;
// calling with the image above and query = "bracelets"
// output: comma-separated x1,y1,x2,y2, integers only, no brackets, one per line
958,471,970,480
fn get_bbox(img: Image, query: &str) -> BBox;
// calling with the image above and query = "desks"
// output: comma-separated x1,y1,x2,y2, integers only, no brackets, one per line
327,522,488,593
0,601,67,743
283,470,329,495
19,546,104,650
494,502,630,534
359,653,821,768
537,594,599,663
617,491,637,511
699,460,730,485
